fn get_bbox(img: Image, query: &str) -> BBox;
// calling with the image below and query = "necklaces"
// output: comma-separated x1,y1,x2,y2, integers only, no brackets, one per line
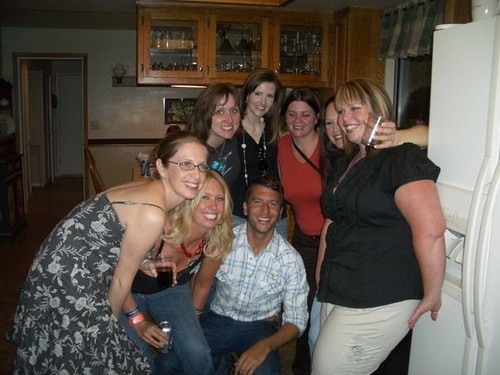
180,237,206,267
243,114,268,186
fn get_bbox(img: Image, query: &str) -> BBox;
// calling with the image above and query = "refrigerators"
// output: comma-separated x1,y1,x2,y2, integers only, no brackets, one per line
409,16,500,375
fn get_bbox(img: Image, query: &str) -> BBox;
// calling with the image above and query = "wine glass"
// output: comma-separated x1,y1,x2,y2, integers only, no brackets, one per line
151,28,187,49
281,31,321,54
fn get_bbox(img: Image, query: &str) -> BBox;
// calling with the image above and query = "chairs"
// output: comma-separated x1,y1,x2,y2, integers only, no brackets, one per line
225,204,296,361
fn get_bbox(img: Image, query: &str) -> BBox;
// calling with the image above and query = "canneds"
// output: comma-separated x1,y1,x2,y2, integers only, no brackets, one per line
141,160,151,176
158,321,173,354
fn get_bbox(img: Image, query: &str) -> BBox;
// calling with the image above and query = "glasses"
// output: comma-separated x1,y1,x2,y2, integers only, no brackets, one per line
167,160,211,173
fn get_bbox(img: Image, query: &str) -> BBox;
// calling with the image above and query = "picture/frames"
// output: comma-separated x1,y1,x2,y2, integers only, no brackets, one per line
164,97,198,124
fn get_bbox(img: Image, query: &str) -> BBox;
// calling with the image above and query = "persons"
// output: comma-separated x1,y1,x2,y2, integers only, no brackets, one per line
189,177,310,375
188,83,244,216
310,95,429,359
310,81,447,375
10,126,210,375
275,89,326,375
230,68,286,242
117,170,236,375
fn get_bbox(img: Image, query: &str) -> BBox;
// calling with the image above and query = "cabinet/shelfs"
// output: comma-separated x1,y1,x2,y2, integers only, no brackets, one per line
0,78,27,241
135,0,386,90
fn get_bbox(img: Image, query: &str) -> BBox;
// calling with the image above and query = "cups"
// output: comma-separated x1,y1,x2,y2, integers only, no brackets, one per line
187,40,194,48
360,112,390,147
151,255,175,272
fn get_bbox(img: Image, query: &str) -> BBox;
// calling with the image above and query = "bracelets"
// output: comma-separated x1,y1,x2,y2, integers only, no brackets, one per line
129,312,144,326
126,306,139,318
195,310,204,314
